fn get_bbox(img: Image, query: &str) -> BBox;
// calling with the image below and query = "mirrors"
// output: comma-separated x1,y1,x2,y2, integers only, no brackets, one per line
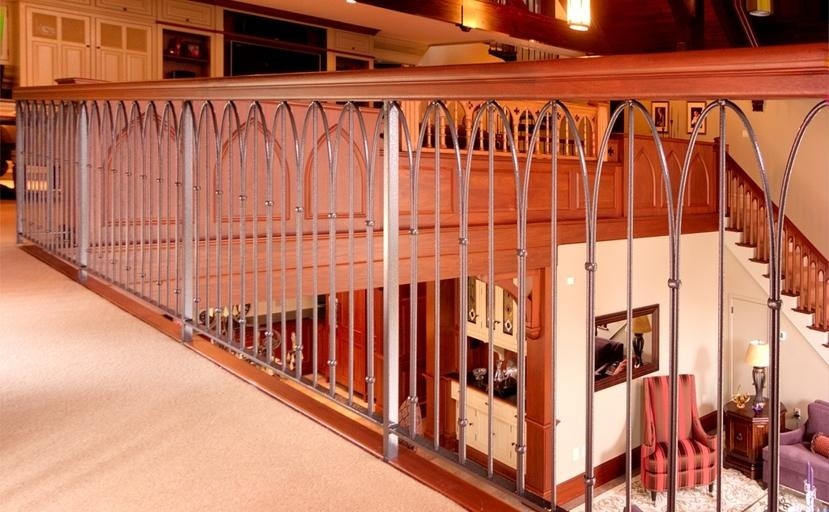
593,303,661,393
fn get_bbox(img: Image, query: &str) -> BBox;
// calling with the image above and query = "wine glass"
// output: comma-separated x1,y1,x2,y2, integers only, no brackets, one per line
473,368,487,381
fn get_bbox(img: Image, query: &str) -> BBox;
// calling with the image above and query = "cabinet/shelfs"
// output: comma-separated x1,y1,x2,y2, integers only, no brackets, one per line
467,273,528,358
724,394,788,490
323,284,426,418
226,317,312,375
449,377,527,484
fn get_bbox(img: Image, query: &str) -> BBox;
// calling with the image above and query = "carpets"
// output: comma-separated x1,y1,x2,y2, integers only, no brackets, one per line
567,466,820,512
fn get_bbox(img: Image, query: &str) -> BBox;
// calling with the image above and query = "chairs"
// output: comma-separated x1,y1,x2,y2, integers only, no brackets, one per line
640,372,718,504
594,335,624,381
759,398,829,505
605,357,631,377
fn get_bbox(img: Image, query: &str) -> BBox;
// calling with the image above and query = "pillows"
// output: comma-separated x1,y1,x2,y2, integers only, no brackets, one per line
810,432,829,459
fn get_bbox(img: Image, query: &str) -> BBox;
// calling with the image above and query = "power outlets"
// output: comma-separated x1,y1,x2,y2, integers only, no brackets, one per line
794,408,800,419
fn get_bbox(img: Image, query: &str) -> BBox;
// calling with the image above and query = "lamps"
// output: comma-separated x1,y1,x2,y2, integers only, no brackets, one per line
744,338,770,405
566,0,592,32
745,0,774,19
629,315,653,369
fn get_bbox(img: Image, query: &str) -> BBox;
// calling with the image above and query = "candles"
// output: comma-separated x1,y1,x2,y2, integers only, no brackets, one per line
809,467,814,492
806,461,811,485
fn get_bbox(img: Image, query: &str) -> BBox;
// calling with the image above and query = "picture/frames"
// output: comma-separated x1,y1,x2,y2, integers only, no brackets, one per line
649,101,670,134
686,100,707,135
